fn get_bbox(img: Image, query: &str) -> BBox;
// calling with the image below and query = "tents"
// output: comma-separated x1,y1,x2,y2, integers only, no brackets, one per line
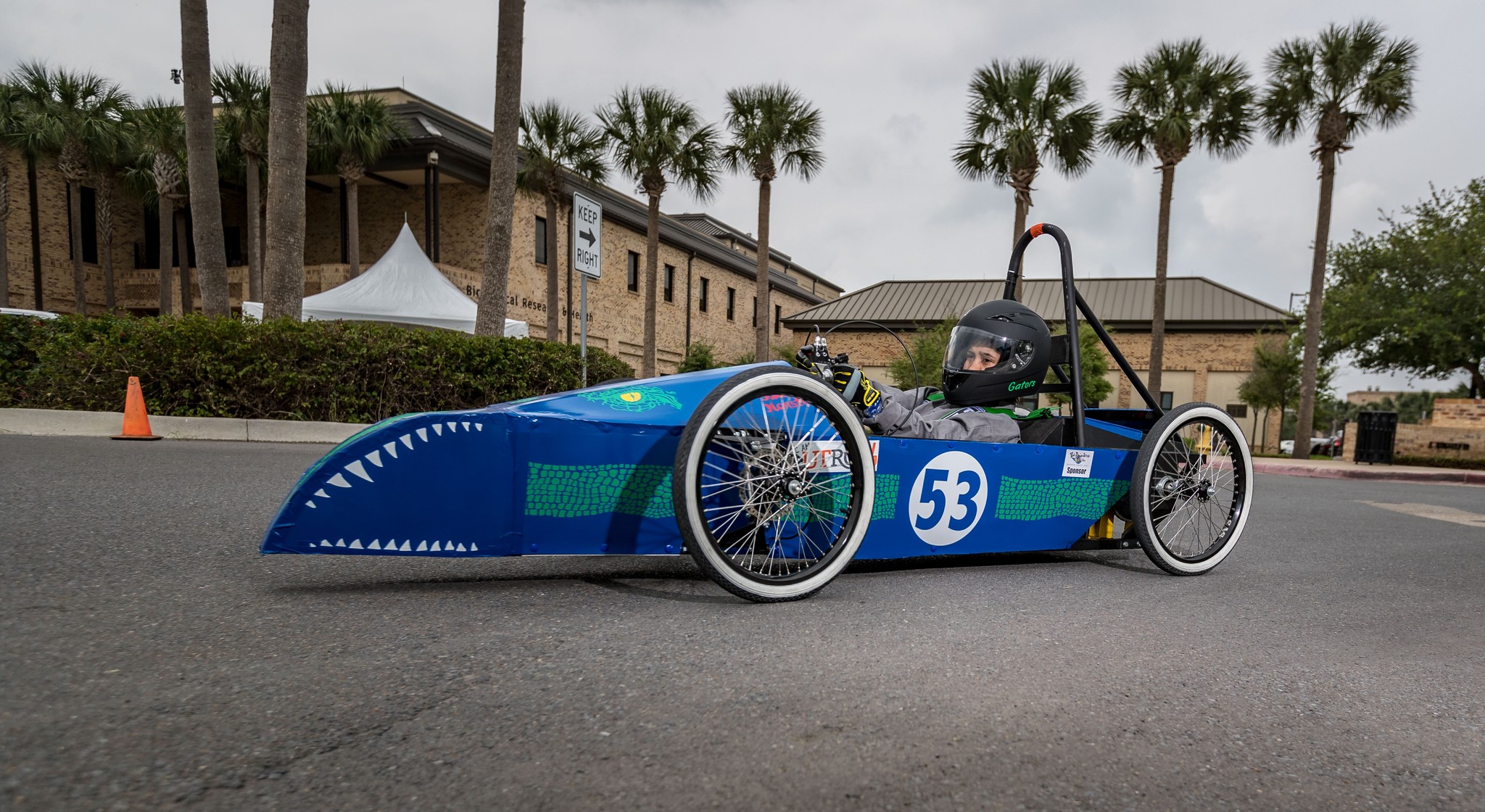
242,212,529,340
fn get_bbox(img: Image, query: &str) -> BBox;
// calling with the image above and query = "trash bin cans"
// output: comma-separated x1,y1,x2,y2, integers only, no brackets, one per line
1353,411,1398,464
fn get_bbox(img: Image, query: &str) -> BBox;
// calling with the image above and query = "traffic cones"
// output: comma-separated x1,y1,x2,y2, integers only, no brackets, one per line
109,377,163,440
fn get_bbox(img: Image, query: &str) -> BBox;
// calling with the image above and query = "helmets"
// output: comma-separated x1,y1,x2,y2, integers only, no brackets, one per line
942,299,1051,407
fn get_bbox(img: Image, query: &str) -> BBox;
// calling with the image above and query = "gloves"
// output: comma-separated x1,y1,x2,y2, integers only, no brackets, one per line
830,363,880,411
796,344,832,375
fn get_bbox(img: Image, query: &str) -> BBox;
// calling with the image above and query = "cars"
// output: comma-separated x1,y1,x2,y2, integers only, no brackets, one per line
1280,431,1341,457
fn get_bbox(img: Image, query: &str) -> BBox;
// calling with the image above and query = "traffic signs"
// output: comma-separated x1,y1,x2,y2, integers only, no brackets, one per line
573,191,602,281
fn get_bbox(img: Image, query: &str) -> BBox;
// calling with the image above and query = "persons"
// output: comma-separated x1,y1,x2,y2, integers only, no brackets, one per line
794,299,1050,444
